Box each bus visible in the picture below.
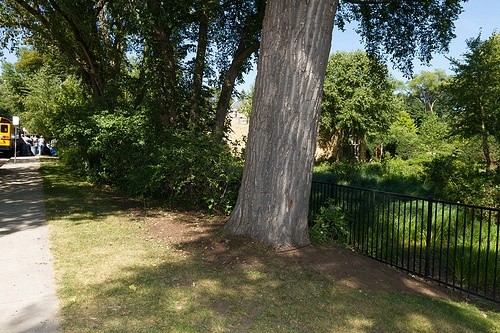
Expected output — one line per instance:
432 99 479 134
0 117 15 158
